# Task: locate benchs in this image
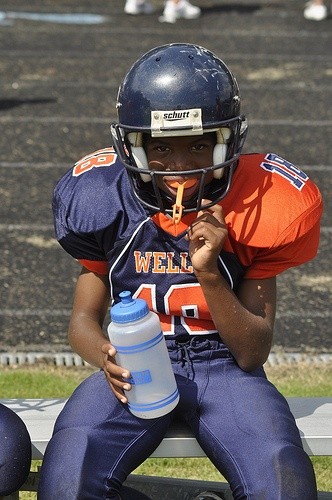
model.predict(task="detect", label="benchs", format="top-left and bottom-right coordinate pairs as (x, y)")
top-left (0, 397), bottom-right (332, 500)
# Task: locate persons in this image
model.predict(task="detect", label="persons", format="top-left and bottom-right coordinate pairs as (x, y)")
top-left (304, 0), bottom-right (328, 20)
top-left (124, 0), bottom-right (201, 19)
top-left (36, 43), bottom-right (323, 500)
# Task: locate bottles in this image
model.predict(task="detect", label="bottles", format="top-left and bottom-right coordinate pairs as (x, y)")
top-left (107, 291), bottom-right (180, 419)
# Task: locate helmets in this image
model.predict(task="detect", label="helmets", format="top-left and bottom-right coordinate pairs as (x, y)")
top-left (110, 42), bottom-right (247, 216)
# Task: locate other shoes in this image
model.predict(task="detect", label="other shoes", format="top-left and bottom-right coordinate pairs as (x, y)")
top-left (163, 1), bottom-right (201, 22)
top-left (303, 4), bottom-right (328, 21)
top-left (123, 0), bottom-right (157, 16)
